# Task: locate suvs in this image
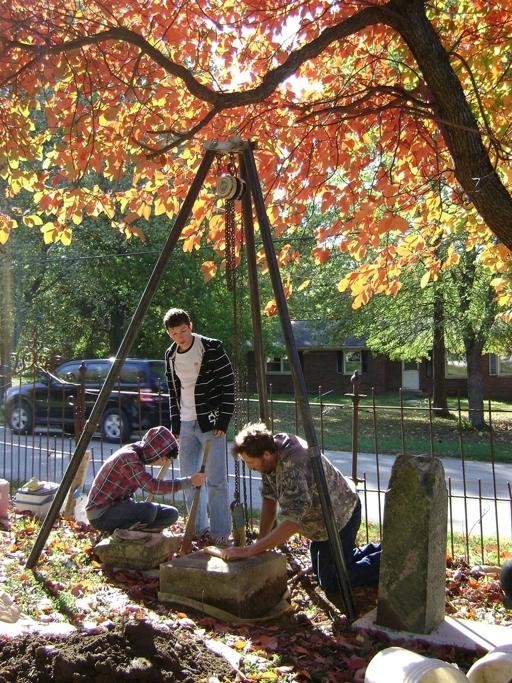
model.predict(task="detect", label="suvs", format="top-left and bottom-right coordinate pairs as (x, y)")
top-left (2, 359), bottom-right (183, 440)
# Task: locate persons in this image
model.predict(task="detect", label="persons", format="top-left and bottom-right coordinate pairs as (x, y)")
top-left (163, 308), bottom-right (234, 546)
top-left (221, 422), bottom-right (381, 593)
top-left (85, 426), bottom-right (207, 540)
